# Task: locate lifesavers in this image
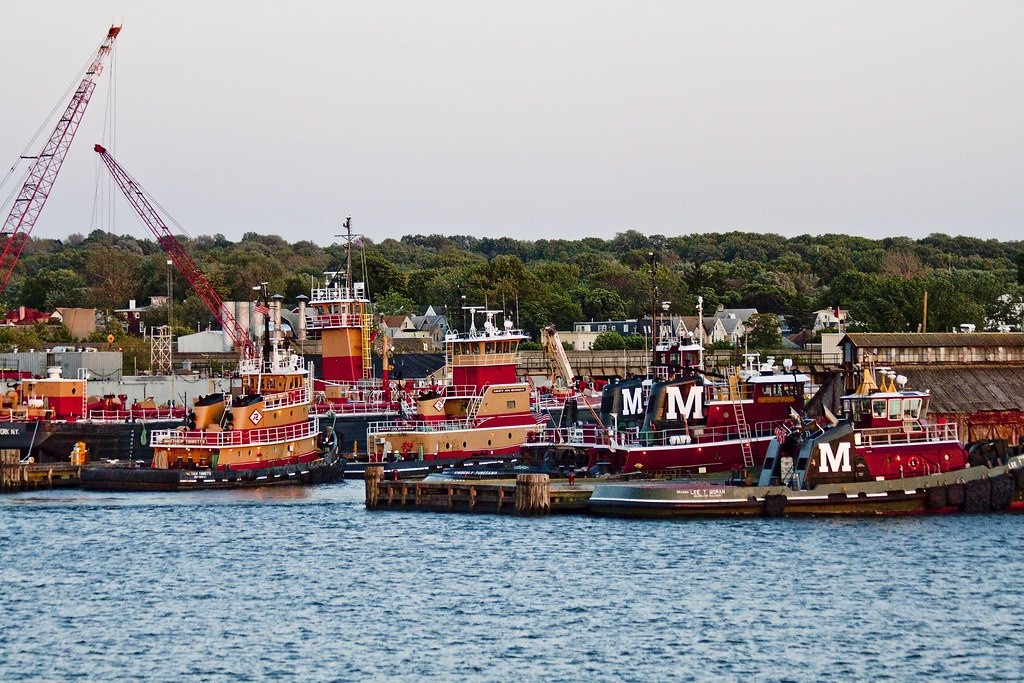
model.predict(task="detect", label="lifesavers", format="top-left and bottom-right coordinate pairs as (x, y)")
top-left (906, 456), bottom-right (920, 470)
top-left (401, 441), bottom-right (411, 452)
top-left (783, 419), bottom-right (794, 428)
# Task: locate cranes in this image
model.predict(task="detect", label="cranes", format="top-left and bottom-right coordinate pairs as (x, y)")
top-left (0, 16), bottom-right (124, 310)
top-left (94, 143), bottom-right (268, 373)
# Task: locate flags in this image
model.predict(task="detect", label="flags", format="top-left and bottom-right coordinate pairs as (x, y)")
top-left (253, 301), bottom-right (270, 314)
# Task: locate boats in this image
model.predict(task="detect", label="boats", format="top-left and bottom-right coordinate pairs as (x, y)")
top-left (0, 206), bottom-right (1024, 519)
